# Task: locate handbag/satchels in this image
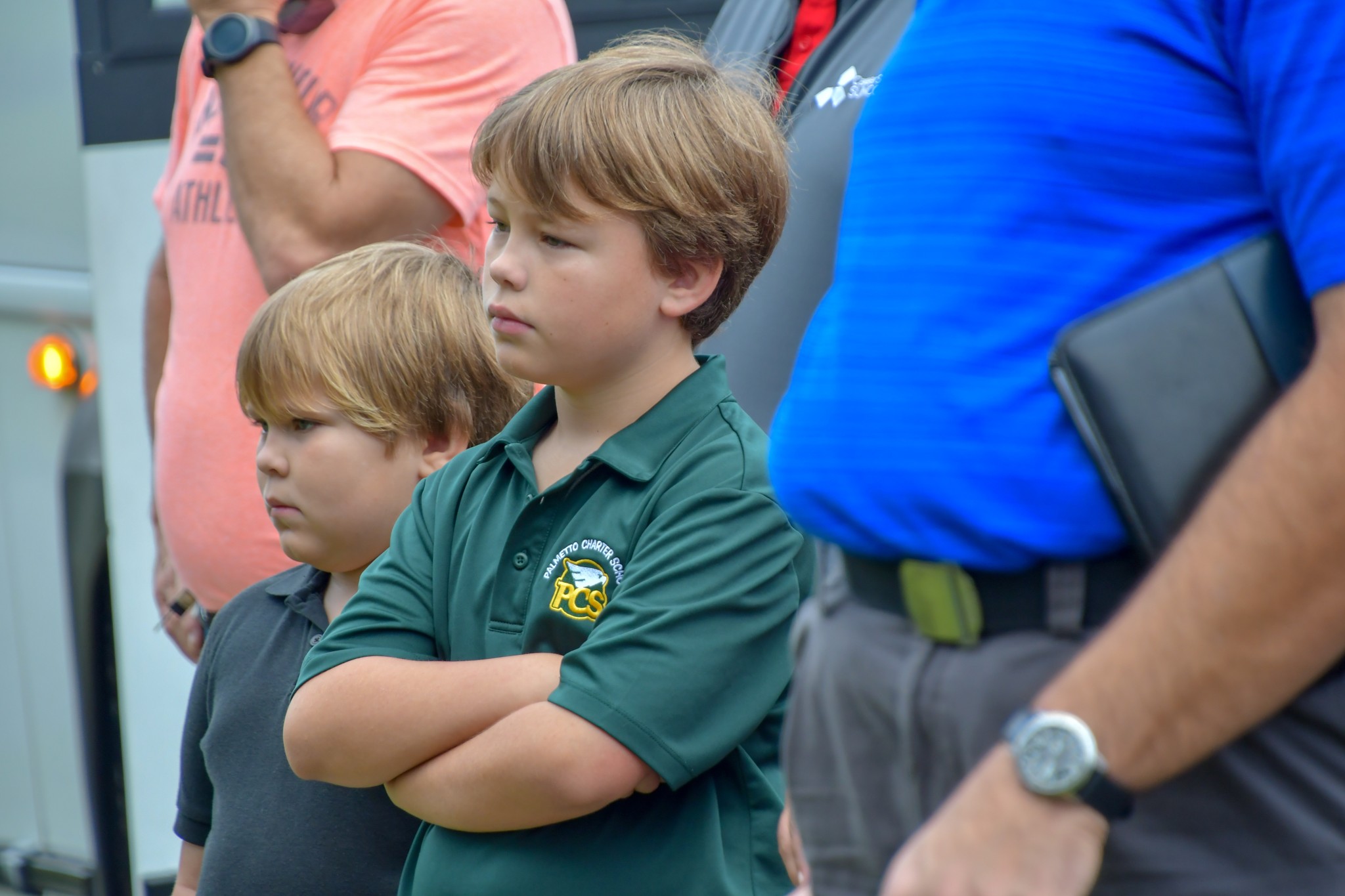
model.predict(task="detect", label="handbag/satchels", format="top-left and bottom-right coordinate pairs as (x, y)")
top-left (1049, 232), bottom-right (1320, 558)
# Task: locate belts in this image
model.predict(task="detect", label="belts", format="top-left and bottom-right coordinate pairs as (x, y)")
top-left (815, 539), bottom-right (1137, 645)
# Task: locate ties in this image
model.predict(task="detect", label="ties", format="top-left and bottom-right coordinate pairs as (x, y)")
top-left (769, 0), bottom-right (836, 118)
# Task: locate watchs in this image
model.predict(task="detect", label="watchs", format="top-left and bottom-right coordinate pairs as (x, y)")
top-left (199, 12), bottom-right (282, 80)
top-left (998, 703), bottom-right (1139, 828)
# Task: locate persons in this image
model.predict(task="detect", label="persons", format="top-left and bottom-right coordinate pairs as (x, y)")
top-left (166, 236), bottom-right (533, 894)
top-left (281, 25), bottom-right (820, 896)
top-left (753, 0), bottom-right (1345, 896)
top-left (136, 0), bottom-right (586, 663)
top-left (686, 0), bottom-right (911, 589)
